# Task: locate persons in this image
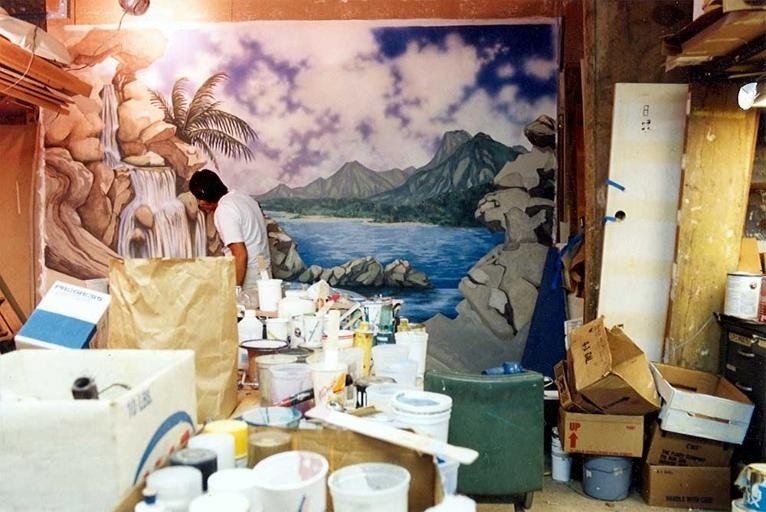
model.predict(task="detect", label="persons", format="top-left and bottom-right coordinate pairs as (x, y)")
top-left (188, 169), bottom-right (273, 289)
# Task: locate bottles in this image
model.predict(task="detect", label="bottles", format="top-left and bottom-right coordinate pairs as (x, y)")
top-left (397, 318), bottom-right (411, 330)
top-left (354, 321), bottom-right (372, 376)
top-left (234, 309), bottom-right (262, 369)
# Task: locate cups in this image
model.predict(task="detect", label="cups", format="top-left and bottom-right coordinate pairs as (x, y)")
top-left (504, 361), bottom-right (520, 375)
top-left (136, 420), bottom-right (411, 511)
top-left (256, 278), bottom-right (283, 310)
top-left (266, 311), bottom-right (341, 344)
top-left (372, 331), bottom-right (452, 389)
top-left (379, 295), bottom-right (392, 333)
top-left (257, 348), bottom-right (348, 407)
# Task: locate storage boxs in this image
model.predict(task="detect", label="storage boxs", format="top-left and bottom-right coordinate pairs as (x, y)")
top-left (556, 316), bottom-right (756, 508)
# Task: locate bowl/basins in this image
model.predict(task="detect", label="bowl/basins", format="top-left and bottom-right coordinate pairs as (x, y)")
top-left (241, 407), bottom-right (302, 430)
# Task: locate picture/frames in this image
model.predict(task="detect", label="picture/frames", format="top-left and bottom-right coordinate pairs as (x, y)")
top-left (45, 2), bottom-right (588, 321)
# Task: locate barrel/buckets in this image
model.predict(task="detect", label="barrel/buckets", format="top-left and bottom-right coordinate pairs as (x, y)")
top-left (724, 271), bottom-right (764, 319)
top-left (582, 455), bottom-right (633, 500)
top-left (253, 277), bottom-right (460, 512)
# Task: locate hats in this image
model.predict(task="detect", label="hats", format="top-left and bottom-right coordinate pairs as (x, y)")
top-left (189, 169), bottom-right (229, 203)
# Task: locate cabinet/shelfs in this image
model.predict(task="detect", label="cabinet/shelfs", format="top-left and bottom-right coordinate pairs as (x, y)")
top-left (712, 312), bottom-right (766, 465)
